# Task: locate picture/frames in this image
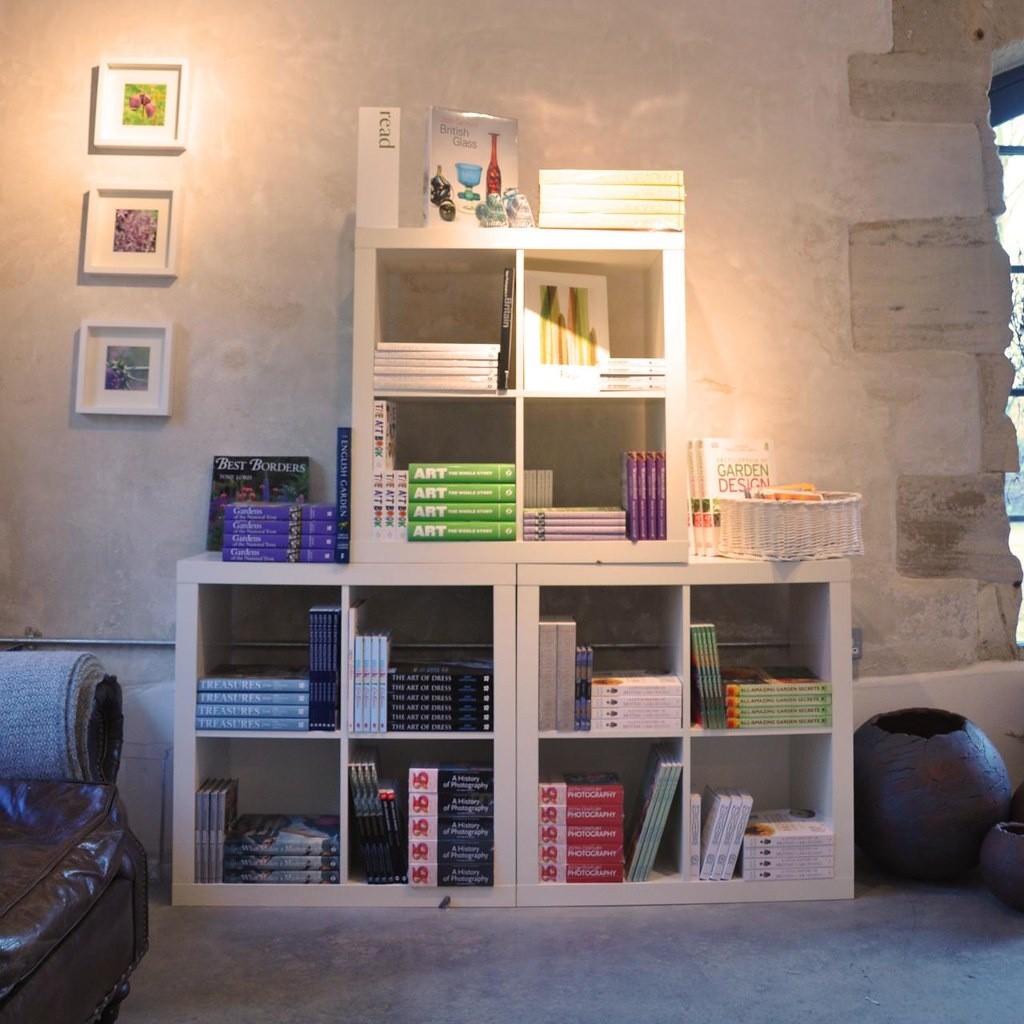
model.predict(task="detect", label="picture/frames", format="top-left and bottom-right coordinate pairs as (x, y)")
top-left (82, 180), bottom-right (180, 278)
top-left (93, 57), bottom-right (190, 152)
top-left (74, 319), bottom-right (173, 416)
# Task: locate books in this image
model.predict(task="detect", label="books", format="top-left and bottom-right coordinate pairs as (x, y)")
top-left (685, 439), bottom-right (779, 557)
top-left (690, 621), bottom-right (833, 730)
top-left (195, 743), bottom-right (684, 886)
top-left (195, 599), bottom-right (681, 733)
top-left (690, 783), bottom-right (835, 882)
top-left (208, 107), bottom-right (684, 566)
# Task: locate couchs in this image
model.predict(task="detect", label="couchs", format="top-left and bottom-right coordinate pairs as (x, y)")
top-left (0, 650), bottom-right (149, 1024)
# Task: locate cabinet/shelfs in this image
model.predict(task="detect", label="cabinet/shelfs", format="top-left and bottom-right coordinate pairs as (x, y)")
top-left (170, 106), bottom-right (854, 907)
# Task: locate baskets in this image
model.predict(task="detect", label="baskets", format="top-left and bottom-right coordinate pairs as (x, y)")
top-left (715, 488), bottom-right (868, 562)
